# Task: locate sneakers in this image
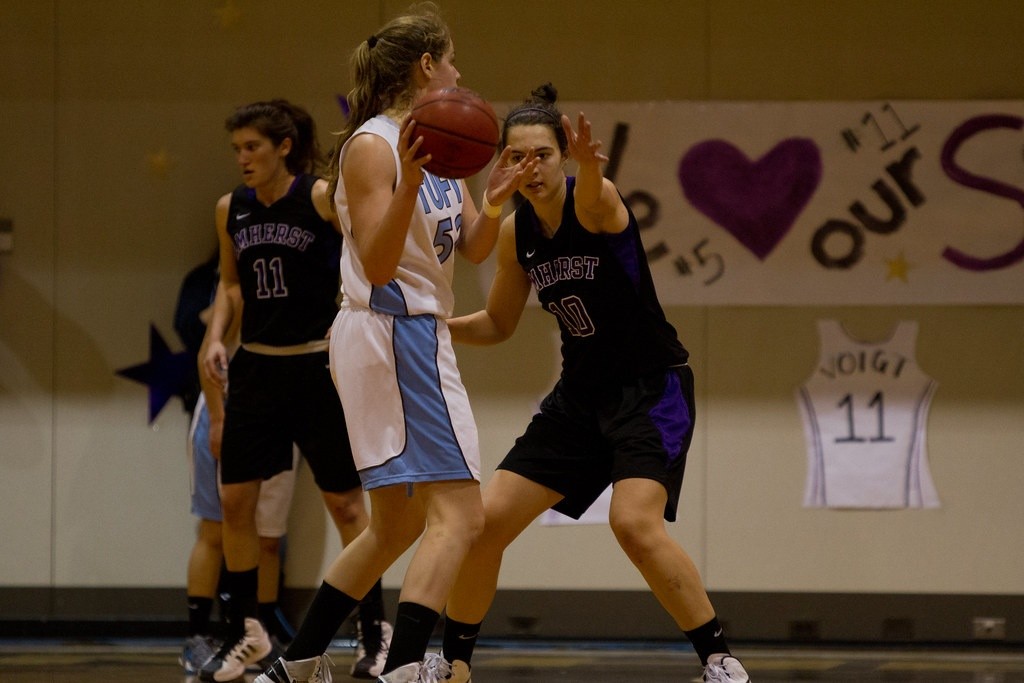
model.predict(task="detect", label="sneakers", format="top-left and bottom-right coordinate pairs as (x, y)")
top-left (377, 648), bottom-right (472, 683)
top-left (214, 617), bottom-right (272, 682)
top-left (253, 650), bottom-right (336, 683)
top-left (347, 612), bottom-right (393, 678)
top-left (177, 635), bottom-right (214, 674)
top-left (700, 653), bottom-right (751, 683)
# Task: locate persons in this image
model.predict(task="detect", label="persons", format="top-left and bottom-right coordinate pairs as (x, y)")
top-left (431, 79), bottom-right (757, 683)
top-left (252, 13), bottom-right (540, 683)
top-left (170, 96), bottom-right (393, 683)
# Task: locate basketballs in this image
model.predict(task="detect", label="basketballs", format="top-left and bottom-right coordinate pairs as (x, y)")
top-left (406, 86), bottom-right (501, 178)
